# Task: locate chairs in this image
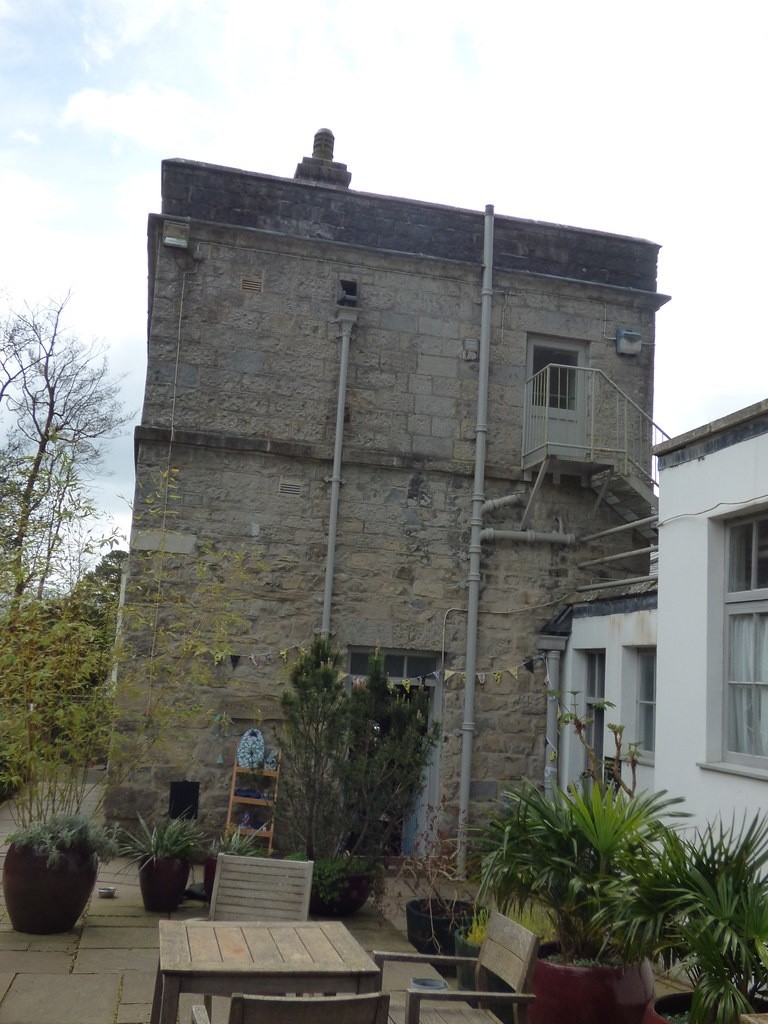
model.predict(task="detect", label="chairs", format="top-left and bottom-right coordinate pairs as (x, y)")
top-left (190, 991), bottom-right (391, 1024)
top-left (371, 908), bottom-right (541, 1024)
top-left (201, 852), bottom-right (315, 1024)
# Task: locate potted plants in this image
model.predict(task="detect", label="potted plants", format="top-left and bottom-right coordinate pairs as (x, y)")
top-left (442, 687), bottom-right (692, 1024)
top-left (450, 894), bottom-right (562, 1024)
top-left (0, 429), bottom-right (312, 931)
top-left (378, 785), bottom-right (488, 979)
top-left (556, 814), bottom-right (768, 1024)
top-left (115, 805), bottom-right (213, 917)
top-left (201, 818), bottom-right (273, 909)
top-left (245, 631), bottom-right (443, 919)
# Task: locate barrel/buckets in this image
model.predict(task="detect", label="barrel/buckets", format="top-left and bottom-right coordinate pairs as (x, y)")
top-left (410, 977), bottom-right (447, 990)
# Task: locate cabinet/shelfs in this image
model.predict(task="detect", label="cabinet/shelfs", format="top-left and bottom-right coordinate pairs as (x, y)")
top-left (223, 743), bottom-right (283, 850)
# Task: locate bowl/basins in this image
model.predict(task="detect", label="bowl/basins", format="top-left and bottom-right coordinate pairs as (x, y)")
top-left (97, 887), bottom-right (117, 898)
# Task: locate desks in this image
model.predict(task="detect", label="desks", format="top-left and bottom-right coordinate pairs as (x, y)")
top-left (151, 919), bottom-right (383, 1024)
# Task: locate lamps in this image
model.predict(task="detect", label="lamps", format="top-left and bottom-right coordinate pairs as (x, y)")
top-left (162, 220), bottom-right (191, 250)
